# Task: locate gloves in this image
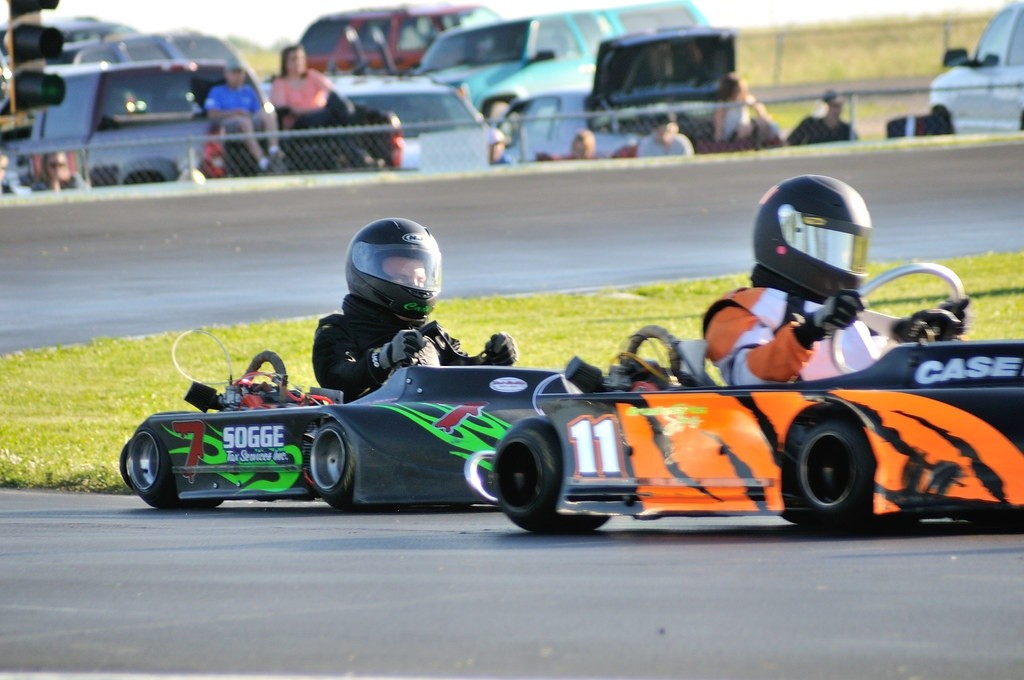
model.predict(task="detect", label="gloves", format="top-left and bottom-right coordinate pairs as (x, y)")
top-left (891, 298), bottom-right (970, 342)
top-left (483, 333), bottom-right (516, 366)
top-left (802, 289), bottom-right (864, 342)
top-left (379, 328), bottom-right (426, 370)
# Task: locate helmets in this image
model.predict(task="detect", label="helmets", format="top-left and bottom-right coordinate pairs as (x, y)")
top-left (345, 217), bottom-right (442, 320)
top-left (752, 174), bottom-right (873, 298)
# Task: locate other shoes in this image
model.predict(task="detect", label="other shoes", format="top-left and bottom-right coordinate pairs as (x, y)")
top-left (263, 161), bottom-right (276, 171)
top-left (270, 150), bottom-right (287, 162)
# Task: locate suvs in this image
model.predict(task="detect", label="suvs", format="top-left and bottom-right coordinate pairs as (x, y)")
top-left (261, 77), bottom-right (508, 169)
top-left (491, 26), bottom-right (786, 167)
top-left (415, 0), bottom-right (712, 120)
top-left (280, 2), bottom-right (503, 72)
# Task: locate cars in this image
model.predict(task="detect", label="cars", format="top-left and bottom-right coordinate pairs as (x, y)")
top-left (44, 17), bottom-right (248, 120)
top-left (928, 0), bottom-right (1023, 132)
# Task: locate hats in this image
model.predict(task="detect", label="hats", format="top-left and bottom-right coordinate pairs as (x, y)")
top-left (484, 126), bottom-right (512, 149)
top-left (226, 58), bottom-right (244, 70)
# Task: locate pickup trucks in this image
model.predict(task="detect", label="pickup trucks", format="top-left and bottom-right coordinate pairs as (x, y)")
top-left (0, 62), bottom-right (274, 190)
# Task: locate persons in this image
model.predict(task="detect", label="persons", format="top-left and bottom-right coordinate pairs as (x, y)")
top-left (30, 147), bottom-right (90, 194)
top-left (702, 173), bottom-right (970, 387)
top-left (313, 216), bottom-right (516, 402)
top-left (451, 76), bottom-right (527, 167)
top-left (532, 119), bottom-right (597, 162)
top-left (635, 112), bottom-right (695, 158)
top-left (714, 74), bottom-right (782, 151)
top-left (786, 88), bottom-right (858, 147)
top-left (206, 43), bottom-right (387, 175)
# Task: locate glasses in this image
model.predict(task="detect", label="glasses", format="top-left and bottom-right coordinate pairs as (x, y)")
top-left (48, 162), bottom-right (66, 169)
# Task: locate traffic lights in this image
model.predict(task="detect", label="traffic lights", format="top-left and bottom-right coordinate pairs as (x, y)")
top-left (8, 0), bottom-right (66, 112)
